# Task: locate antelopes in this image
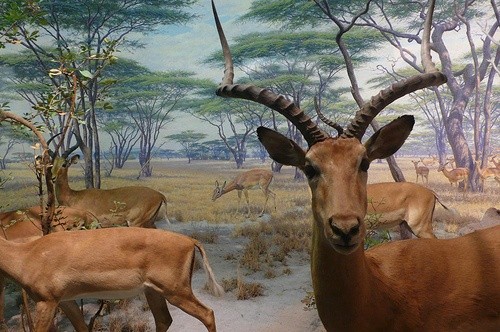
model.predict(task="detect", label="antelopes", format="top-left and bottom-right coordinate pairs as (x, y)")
top-left (364, 183), bottom-right (449, 241)
top-left (410, 152), bottom-right (499, 191)
top-left (211, 1), bottom-right (499, 332)
top-left (0, 228), bottom-right (223, 332)
top-left (33, 131), bottom-right (173, 231)
top-left (0, 205), bottom-right (103, 242)
top-left (212, 169), bottom-right (277, 219)
